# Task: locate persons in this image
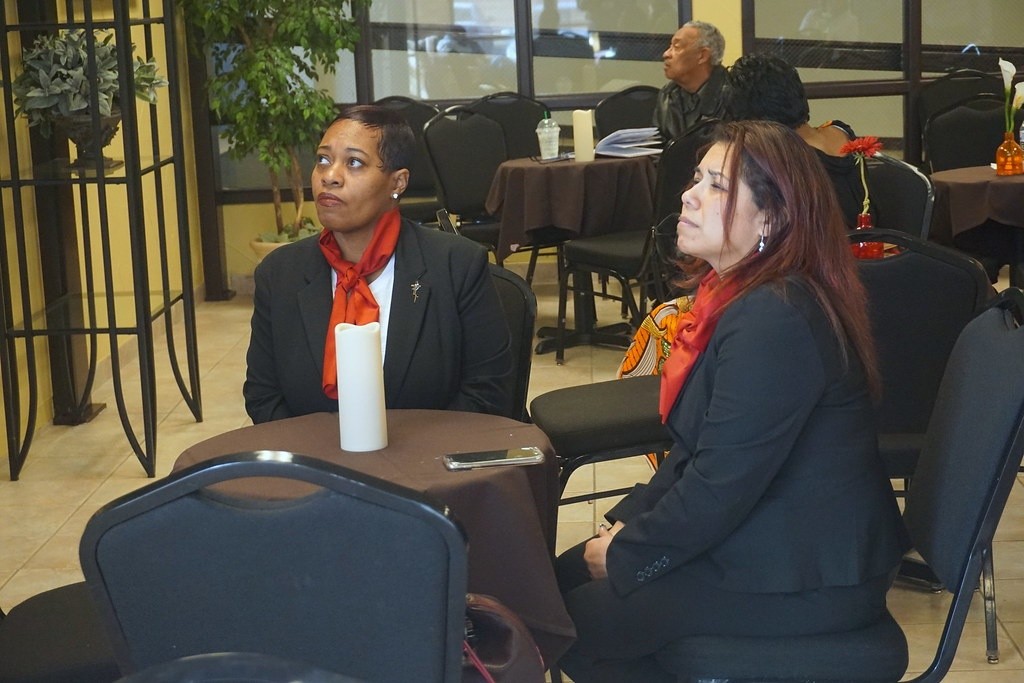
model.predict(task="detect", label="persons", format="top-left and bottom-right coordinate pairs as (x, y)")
top-left (242, 105), bottom-right (516, 416)
top-left (553, 119), bottom-right (910, 683)
top-left (722, 52), bottom-right (878, 228)
top-left (652, 20), bottom-right (730, 144)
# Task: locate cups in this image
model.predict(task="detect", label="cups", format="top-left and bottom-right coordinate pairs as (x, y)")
top-left (534, 119), bottom-right (561, 160)
top-left (571, 109), bottom-right (595, 162)
top-left (334, 321), bottom-right (388, 452)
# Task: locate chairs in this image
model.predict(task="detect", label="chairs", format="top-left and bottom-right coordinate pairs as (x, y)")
top-left (0, 81), bottom-right (1024, 683)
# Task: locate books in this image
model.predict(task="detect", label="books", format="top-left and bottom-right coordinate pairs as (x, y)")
top-left (567, 126), bottom-right (663, 158)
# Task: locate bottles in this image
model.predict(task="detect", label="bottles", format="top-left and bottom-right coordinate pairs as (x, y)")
top-left (1019, 121), bottom-right (1024, 150)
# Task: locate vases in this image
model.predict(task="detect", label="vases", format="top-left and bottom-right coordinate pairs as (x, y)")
top-left (847, 215), bottom-right (884, 261)
top-left (996, 132), bottom-right (1024, 176)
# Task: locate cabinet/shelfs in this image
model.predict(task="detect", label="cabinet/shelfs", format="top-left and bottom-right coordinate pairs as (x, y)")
top-left (0, 0), bottom-right (204, 480)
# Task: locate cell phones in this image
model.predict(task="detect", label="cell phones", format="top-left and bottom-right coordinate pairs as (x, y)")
top-left (443, 447), bottom-right (545, 471)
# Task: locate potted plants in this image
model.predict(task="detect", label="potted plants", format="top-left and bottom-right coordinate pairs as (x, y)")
top-left (13, 33), bottom-right (166, 176)
top-left (188, 0), bottom-right (379, 262)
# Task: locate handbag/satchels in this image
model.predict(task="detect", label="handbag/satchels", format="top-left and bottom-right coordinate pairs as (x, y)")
top-left (463, 591), bottom-right (545, 682)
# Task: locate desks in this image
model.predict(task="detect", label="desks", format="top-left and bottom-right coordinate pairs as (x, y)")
top-left (170, 401), bottom-right (579, 683)
top-left (930, 161), bottom-right (1024, 289)
top-left (486, 149), bottom-right (660, 362)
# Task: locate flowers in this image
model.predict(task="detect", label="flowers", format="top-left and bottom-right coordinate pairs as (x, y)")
top-left (837, 134), bottom-right (885, 214)
top-left (989, 57), bottom-right (1024, 135)
